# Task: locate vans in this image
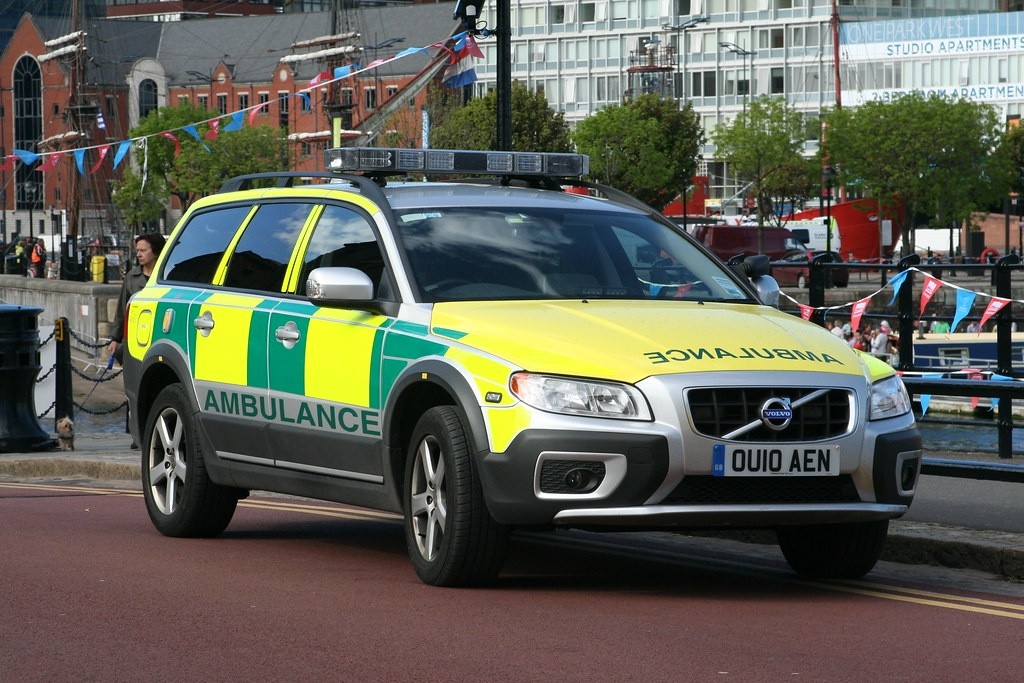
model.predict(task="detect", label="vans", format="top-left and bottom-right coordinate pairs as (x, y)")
top-left (740, 215), bottom-right (843, 261)
top-left (690, 225), bottom-right (815, 289)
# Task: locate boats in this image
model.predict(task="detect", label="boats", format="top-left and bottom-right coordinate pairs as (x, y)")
top-left (889, 330), bottom-right (1024, 420)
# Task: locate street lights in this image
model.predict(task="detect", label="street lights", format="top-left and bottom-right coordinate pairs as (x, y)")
top-left (822, 168), bottom-right (836, 255)
top-left (48, 204), bottom-right (56, 263)
top-left (24, 181), bottom-right (36, 243)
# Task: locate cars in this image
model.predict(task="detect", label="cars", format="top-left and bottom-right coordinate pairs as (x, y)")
top-left (768, 249), bottom-right (849, 287)
top-left (99, 235), bottom-right (132, 251)
top-left (120, 146), bottom-right (925, 589)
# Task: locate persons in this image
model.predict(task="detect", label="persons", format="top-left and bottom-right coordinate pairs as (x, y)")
top-left (105, 233), bottom-right (167, 451)
top-left (823, 311), bottom-right (1020, 373)
top-left (0, 234), bottom-right (132, 278)
